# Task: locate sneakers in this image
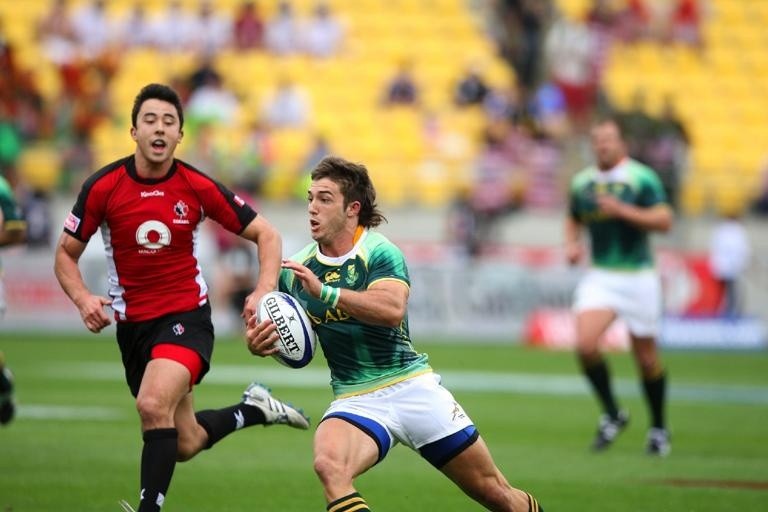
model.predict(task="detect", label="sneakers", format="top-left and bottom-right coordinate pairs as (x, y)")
top-left (591, 407), bottom-right (629, 451)
top-left (242, 379), bottom-right (313, 432)
top-left (644, 425), bottom-right (673, 459)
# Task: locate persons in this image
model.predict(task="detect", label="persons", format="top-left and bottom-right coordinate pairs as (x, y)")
top-left (55, 84), bottom-right (310, 512)
top-left (246, 157), bottom-right (541, 511)
top-left (1, 0), bottom-right (768, 324)
top-left (703, 209), bottom-right (753, 322)
top-left (0, 174), bottom-right (27, 426)
top-left (565, 114), bottom-right (672, 458)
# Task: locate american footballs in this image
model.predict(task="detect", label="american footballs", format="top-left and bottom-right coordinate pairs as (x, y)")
top-left (257, 291), bottom-right (316, 369)
